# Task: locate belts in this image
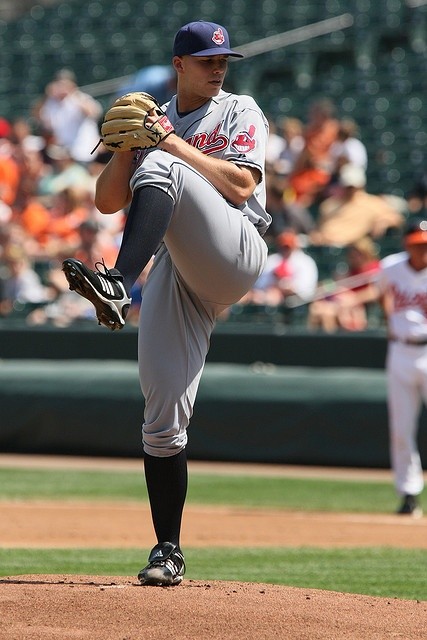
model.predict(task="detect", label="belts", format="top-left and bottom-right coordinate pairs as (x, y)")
top-left (389, 334), bottom-right (426, 348)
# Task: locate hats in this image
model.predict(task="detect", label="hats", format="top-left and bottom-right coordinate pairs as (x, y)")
top-left (277, 231), bottom-right (293, 248)
top-left (174, 18), bottom-right (244, 60)
top-left (403, 217), bottom-right (427, 245)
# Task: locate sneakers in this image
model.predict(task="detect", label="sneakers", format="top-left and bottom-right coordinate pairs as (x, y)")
top-left (137, 541), bottom-right (186, 586)
top-left (61, 258), bottom-right (132, 331)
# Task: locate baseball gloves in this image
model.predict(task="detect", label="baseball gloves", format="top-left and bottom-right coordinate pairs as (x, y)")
top-left (90, 91), bottom-right (175, 154)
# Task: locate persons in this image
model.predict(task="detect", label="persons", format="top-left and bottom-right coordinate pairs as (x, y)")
top-left (337, 220), bottom-right (427, 518)
top-left (245, 106), bottom-right (405, 331)
top-left (64, 17), bottom-right (273, 588)
top-left (0, 71), bottom-right (151, 331)
